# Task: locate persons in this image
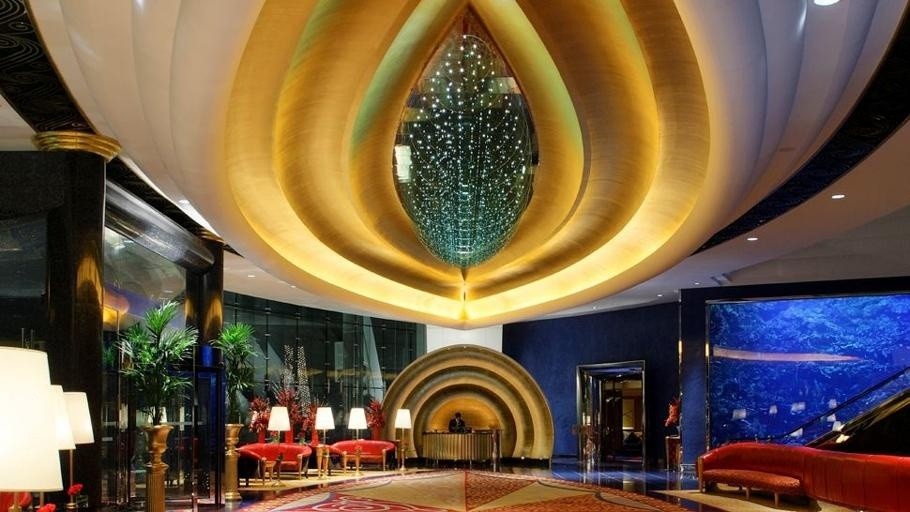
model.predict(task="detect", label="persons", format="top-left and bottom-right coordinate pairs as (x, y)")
top-left (448, 412), bottom-right (465, 434)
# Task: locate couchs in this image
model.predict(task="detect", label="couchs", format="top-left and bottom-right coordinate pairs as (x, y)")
top-left (236, 439), bottom-right (397, 489)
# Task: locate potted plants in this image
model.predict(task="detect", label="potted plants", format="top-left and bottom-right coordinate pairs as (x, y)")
top-left (111, 296), bottom-right (200, 511)
top-left (206, 321), bottom-right (260, 502)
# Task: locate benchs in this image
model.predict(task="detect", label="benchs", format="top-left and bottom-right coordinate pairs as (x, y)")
top-left (702, 468), bottom-right (802, 507)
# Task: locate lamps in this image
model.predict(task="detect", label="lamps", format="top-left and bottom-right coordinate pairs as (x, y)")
top-left (265, 405), bottom-right (412, 488)
